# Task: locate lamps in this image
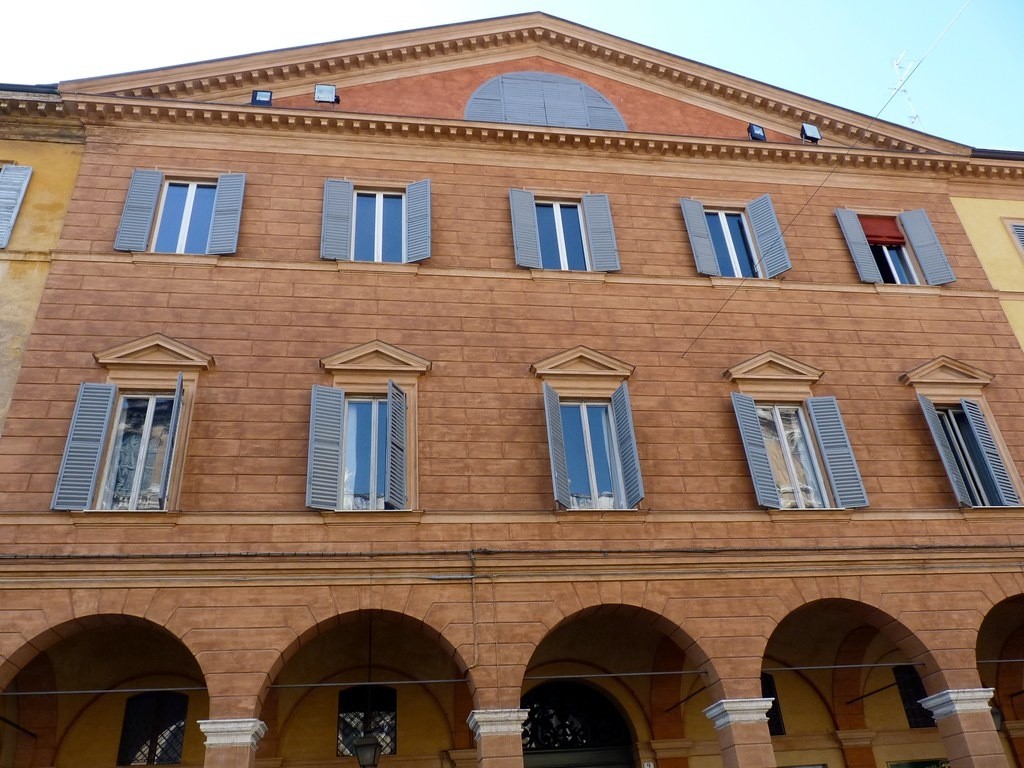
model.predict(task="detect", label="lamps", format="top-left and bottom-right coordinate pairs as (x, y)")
top-left (354, 620), bottom-right (382, 768)
top-left (798, 124), bottom-right (820, 145)
top-left (748, 122), bottom-right (767, 140)
top-left (314, 83), bottom-right (341, 112)
top-left (252, 90), bottom-right (272, 106)
